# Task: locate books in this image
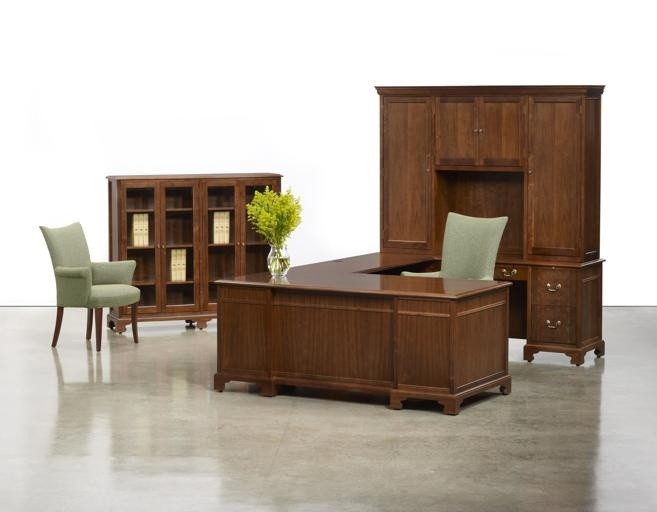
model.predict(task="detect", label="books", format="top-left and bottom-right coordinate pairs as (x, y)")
top-left (170, 249), bottom-right (187, 282)
top-left (131, 213), bottom-right (149, 247)
top-left (213, 211), bottom-right (230, 244)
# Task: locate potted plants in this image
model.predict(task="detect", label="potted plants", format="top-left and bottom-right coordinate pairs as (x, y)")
top-left (245, 184), bottom-right (304, 278)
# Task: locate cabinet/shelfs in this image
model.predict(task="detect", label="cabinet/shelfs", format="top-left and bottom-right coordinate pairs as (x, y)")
top-left (373, 86), bottom-right (606, 366)
top-left (213, 252), bottom-right (514, 415)
top-left (105, 173), bottom-right (284, 335)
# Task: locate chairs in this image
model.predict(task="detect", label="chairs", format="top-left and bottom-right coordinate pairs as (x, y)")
top-left (38, 222), bottom-right (141, 352)
top-left (400, 210), bottom-right (510, 281)
